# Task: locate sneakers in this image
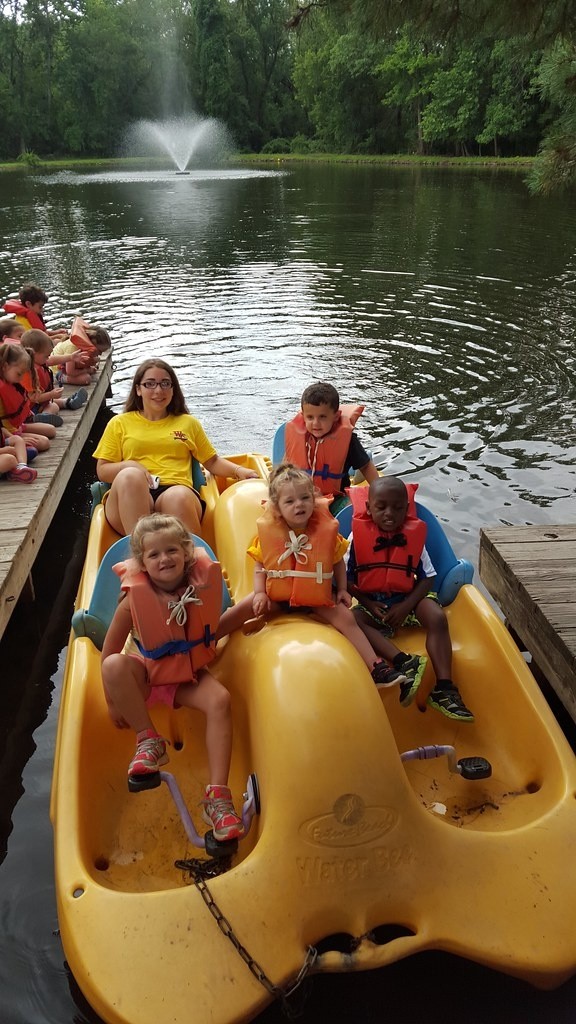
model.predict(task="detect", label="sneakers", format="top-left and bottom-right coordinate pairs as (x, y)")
top-left (425, 685), bottom-right (474, 721)
top-left (127, 729), bottom-right (171, 776)
top-left (198, 784), bottom-right (246, 841)
top-left (371, 658), bottom-right (408, 690)
top-left (396, 654), bottom-right (427, 706)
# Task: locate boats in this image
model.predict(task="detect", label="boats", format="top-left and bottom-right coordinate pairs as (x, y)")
top-left (44, 451), bottom-right (576, 1024)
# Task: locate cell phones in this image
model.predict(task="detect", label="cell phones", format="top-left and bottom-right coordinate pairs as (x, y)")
top-left (148, 475), bottom-right (159, 489)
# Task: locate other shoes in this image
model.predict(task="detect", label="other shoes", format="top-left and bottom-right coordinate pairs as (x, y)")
top-left (53, 370), bottom-right (64, 385)
top-left (26, 447), bottom-right (38, 460)
top-left (65, 387), bottom-right (88, 410)
top-left (6, 465), bottom-right (37, 483)
top-left (33, 413), bottom-right (64, 427)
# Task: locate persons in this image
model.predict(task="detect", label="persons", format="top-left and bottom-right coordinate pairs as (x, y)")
top-left (0, 282), bottom-right (111, 484)
top-left (345, 476), bottom-right (475, 721)
top-left (100, 513), bottom-right (245, 839)
top-left (91, 359), bottom-right (260, 538)
top-left (214, 464), bottom-right (407, 690)
top-left (281, 382), bottom-right (380, 513)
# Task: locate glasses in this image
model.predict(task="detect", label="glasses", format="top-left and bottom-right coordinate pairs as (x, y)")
top-left (139, 381), bottom-right (173, 389)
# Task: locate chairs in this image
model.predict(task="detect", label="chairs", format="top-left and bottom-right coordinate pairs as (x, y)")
top-left (333, 499), bottom-right (473, 607)
top-left (72, 531), bottom-right (234, 652)
top-left (273, 420), bottom-right (374, 487)
